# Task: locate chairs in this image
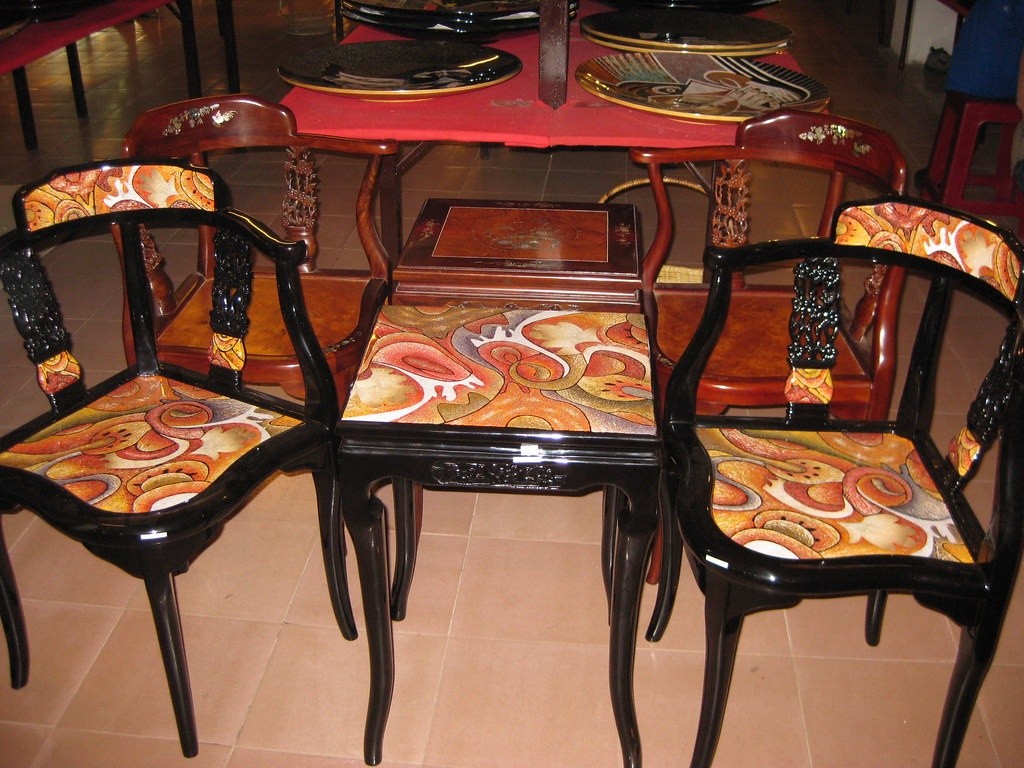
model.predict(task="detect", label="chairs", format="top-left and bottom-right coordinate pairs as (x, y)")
top-left (0, 94), bottom-right (1024, 768)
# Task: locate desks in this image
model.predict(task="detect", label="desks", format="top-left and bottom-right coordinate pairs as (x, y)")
top-left (0, 0), bottom-right (239, 151)
top-left (279, 1), bottom-right (828, 281)
top-left (351, 305), bottom-right (668, 768)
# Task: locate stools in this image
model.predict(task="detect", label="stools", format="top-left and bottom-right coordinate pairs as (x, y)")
top-left (922, 91), bottom-right (1024, 244)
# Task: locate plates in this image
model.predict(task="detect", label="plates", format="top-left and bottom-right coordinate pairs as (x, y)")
top-left (578, 0), bottom-right (793, 56)
top-left (575, 53), bottom-right (832, 123)
top-left (274, 44), bottom-right (523, 103)
top-left (340, 0), bottom-right (581, 42)
